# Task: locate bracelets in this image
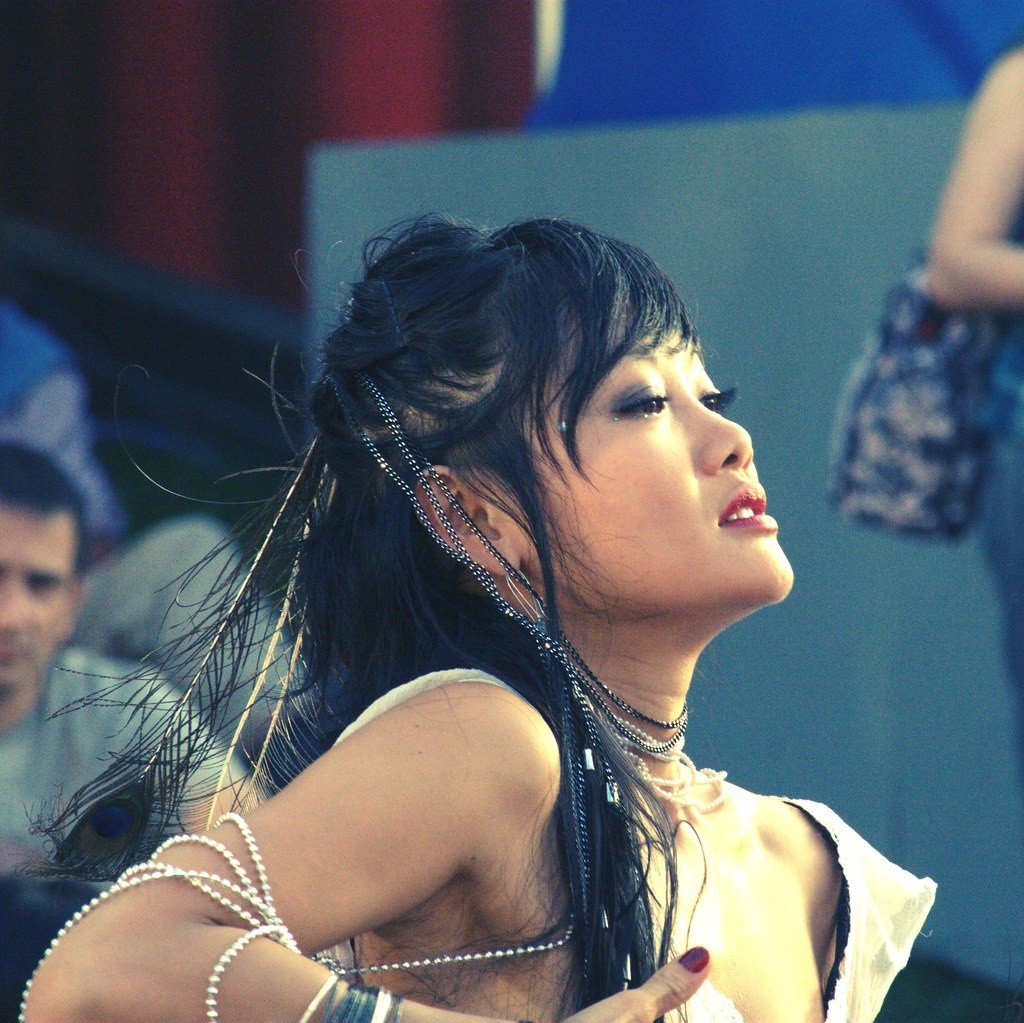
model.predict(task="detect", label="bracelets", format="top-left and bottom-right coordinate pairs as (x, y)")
top-left (364, 984), bottom-right (397, 1021)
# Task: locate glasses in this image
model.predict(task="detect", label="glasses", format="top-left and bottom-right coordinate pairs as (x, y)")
top-left (1, 562), bottom-right (68, 602)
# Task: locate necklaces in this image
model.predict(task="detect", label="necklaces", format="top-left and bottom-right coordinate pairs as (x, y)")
top-left (573, 718), bottom-right (727, 827)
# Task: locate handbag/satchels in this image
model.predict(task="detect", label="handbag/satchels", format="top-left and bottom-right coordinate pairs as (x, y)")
top-left (816, 236), bottom-right (1007, 547)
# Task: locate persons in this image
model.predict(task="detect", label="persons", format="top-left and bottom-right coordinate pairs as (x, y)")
top-left (1, 440), bottom-right (270, 870)
top-left (835, 35), bottom-right (1021, 778)
top-left (4, 294), bottom-right (118, 575)
top-left (22, 209), bottom-right (938, 1023)
top-left (6, 879), bottom-right (100, 1020)
